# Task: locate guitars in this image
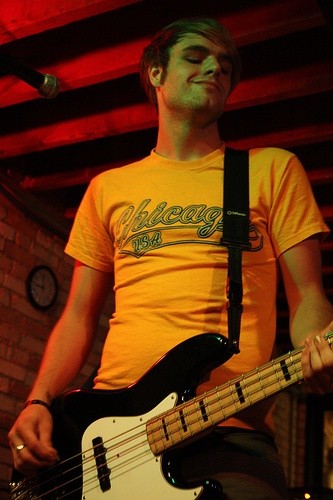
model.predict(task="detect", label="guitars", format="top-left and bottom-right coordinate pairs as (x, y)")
top-left (8, 331), bottom-right (333, 500)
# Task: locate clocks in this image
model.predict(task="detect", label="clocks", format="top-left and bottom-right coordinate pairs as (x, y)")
top-left (26, 264), bottom-right (58, 312)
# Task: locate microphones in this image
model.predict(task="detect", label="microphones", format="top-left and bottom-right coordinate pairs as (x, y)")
top-left (0, 53), bottom-right (60, 101)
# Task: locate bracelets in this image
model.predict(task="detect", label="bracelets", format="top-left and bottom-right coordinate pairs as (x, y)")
top-left (25, 400), bottom-right (49, 410)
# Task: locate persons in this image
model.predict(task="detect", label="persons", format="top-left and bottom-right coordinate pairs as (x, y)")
top-left (8, 18), bottom-right (332, 500)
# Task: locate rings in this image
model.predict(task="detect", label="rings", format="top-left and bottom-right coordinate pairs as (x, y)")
top-left (16, 444), bottom-right (27, 453)
